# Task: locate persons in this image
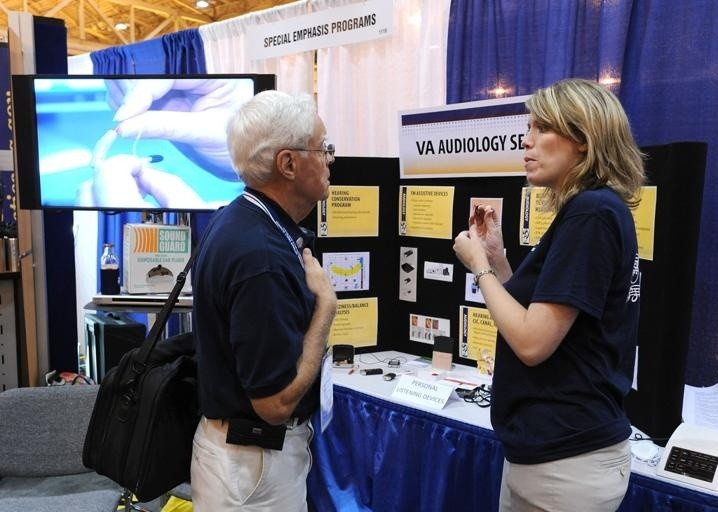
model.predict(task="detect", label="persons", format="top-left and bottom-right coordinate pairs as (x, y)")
top-left (452, 77), bottom-right (642, 510)
top-left (77, 77), bottom-right (254, 209)
top-left (190, 90), bottom-right (339, 512)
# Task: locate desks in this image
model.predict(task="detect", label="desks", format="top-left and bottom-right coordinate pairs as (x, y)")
top-left (311, 348), bottom-right (718, 512)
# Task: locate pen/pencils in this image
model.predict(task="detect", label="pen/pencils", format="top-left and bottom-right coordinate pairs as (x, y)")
top-left (348, 364), bottom-right (359, 374)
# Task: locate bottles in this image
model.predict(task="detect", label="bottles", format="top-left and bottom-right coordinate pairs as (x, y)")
top-left (100, 244), bottom-right (121, 295)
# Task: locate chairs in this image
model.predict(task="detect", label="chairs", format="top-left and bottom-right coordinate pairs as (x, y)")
top-left (0, 384), bottom-right (123, 512)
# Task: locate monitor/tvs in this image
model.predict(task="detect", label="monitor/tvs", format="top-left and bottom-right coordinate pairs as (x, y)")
top-left (12, 73), bottom-right (276, 212)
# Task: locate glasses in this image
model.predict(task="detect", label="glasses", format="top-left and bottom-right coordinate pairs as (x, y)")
top-left (276, 143), bottom-right (335, 162)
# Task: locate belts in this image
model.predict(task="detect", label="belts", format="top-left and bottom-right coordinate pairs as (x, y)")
top-left (288, 415), bottom-right (309, 427)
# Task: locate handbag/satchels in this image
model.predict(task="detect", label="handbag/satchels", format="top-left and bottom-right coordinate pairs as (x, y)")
top-left (81, 331), bottom-right (200, 503)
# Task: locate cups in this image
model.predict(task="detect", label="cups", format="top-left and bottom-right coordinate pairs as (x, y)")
top-left (332, 344), bottom-right (355, 368)
top-left (0, 235), bottom-right (21, 272)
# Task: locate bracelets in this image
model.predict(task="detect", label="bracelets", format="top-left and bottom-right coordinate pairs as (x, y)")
top-left (474, 270), bottom-right (496, 286)
